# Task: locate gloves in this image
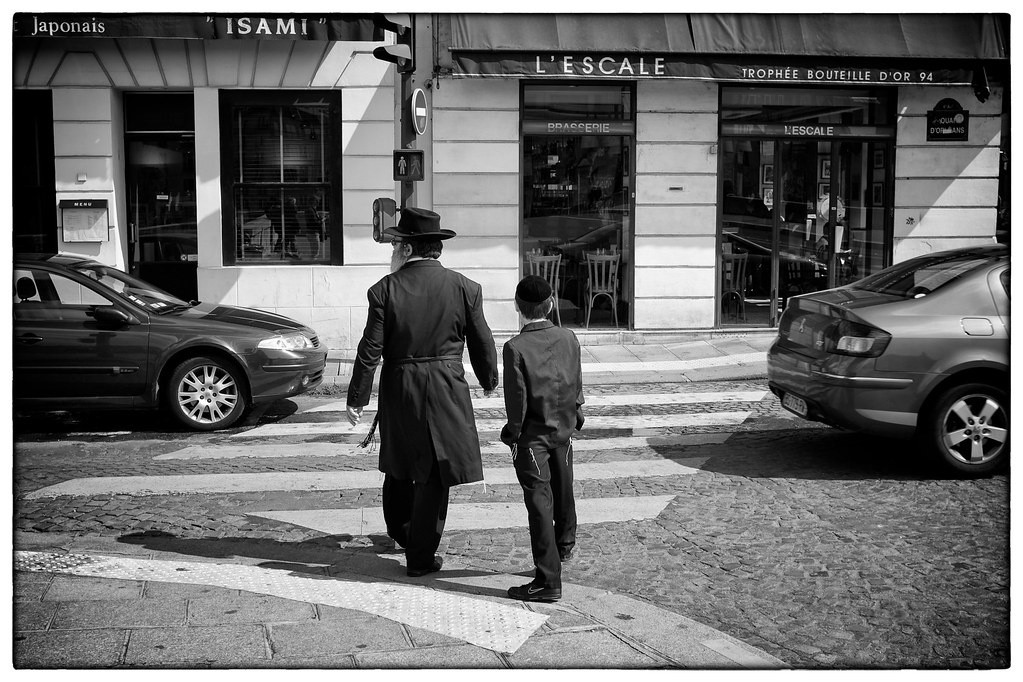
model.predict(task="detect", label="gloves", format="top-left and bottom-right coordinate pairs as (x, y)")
top-left (484, 387), bottom-right (497, 397)
top-left (346, 405), bottom-right (364, 427)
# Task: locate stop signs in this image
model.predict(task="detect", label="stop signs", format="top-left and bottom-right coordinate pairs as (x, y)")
top-left (412, 87), bottom-right (428, 135)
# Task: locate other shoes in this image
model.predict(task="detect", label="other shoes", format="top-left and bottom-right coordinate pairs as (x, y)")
top-left (407, 556), bottom-right (443, 577)
top-left (387, 527), bottom-right (407, 548)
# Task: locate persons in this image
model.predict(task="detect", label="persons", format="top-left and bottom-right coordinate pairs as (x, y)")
top-left (501, 275), bottom-right (584, 602)
top-left (346, 207), bottom-right (498, 576)
top-left (267, 196), bottom-right (324, 260)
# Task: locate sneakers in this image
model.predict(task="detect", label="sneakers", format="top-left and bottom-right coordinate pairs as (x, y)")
top-left (560, 551), bottom-right (573, 562)
top-left (508, 581), bottom-right (562, 602)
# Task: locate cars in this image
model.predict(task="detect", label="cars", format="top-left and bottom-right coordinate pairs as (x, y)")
top-left (765, 239), bottom-right (1012, 473)
top-left (536, 220), bottom-right (832, 325)
top-left (127, 230), bottom-right (269, 263)
top-left (12, 250), bottom-right (330, 432)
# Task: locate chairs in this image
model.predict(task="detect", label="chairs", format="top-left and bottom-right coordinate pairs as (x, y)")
top-left (16, 277), bottom-right (49, 321)
top-left (779, 248), bottom-right (860, 313)
top-left (526, 254), bottom-right (563, 329)
top-left (582, 248), bottom-right (620, 331)
top-left (721, 253), bottom-right (748, 324)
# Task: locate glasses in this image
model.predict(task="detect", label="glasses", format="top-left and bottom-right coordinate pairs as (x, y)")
top-left (390, 238), bottom-right (404, 246)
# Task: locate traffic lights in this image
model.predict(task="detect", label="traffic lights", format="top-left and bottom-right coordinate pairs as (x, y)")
top-left (372, 13), bottom-right (414, 73)
top-left (392, 149), bottom-right (425, 181)
top-left (373, 197), bottom-right (395, 243)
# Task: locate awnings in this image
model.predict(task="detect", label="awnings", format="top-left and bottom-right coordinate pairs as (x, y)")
top-left (451, 13), bottom-right (1008, 85)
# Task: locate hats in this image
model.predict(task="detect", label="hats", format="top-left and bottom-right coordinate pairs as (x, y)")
top-left (381, 207), bottom-right (456, 239)
top-left (517, 275), bottom-right (552, 302)
